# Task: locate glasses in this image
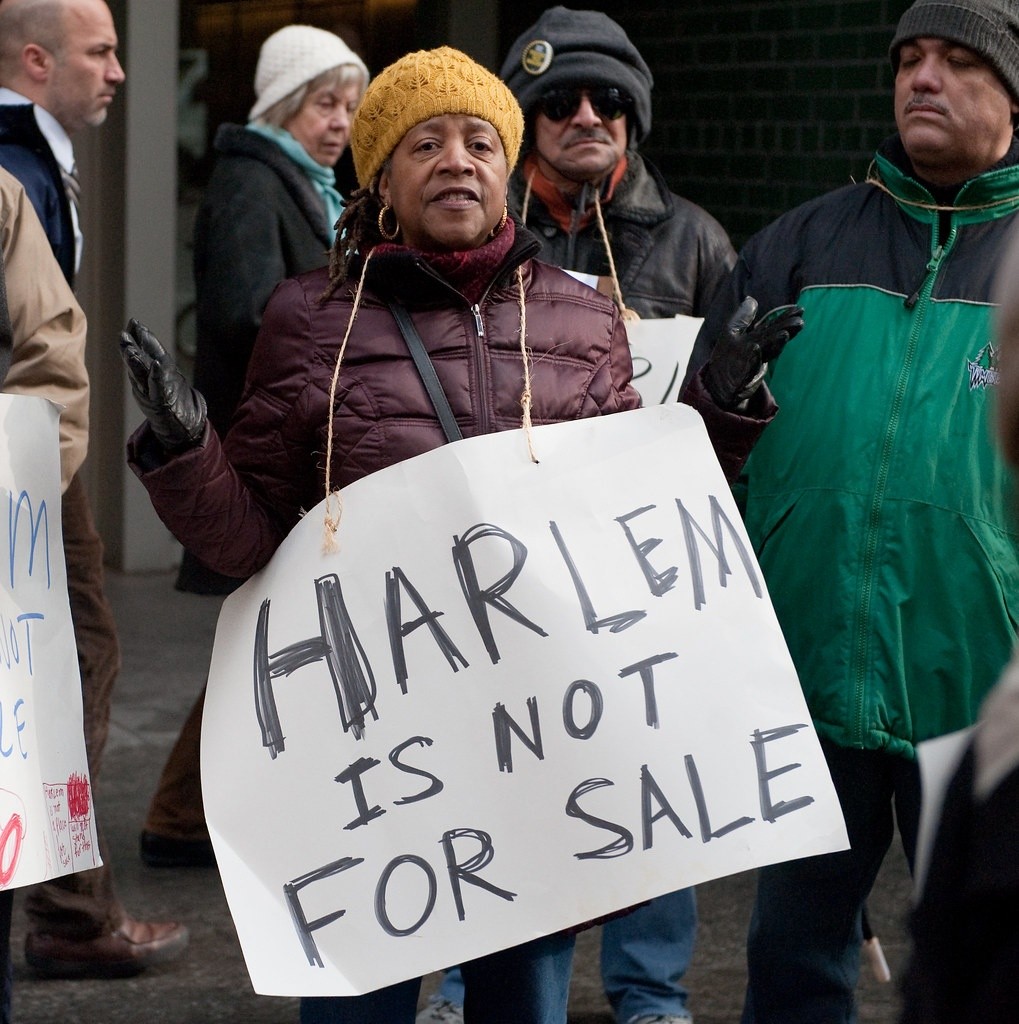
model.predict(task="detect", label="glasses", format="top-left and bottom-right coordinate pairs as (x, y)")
top-left (538, 83), bottom-right (634, 120)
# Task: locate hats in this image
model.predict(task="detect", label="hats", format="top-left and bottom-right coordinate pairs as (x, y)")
top-left (500, 7), bottom-right (655, 150)
top-left (248, 25), bottom-right (369, 121)
top-left (351, 46), bottom-right (524, 190)
top-left (889, 0), bottom-right (1019, 102)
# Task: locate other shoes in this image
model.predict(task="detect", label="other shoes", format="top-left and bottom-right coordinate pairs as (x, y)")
top-left (26, 916), bottom-right (188, 979)
top-left (141, 831), bottom-right (218, 869)
top-left (413, 995), bottom-right (464, 1024)
top-left (632, 1015), bottom-right (693, 1024)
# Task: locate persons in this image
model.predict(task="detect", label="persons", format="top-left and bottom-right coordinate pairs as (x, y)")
top-left (134, 22), bottom-right (371, 868)
top-left (500, 0), bottom-right (756, 1023)
top-left (678, 0), bottom-right (1019, 1023)
top-left (1, 167), bottom-right (92, 1023)
top-left (118, 47), bottom-right (645, 1022)
top-left (0, 0), bottom-right (188, 982)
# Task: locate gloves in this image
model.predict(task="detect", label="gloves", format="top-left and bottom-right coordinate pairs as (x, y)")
top-left (116, 318), bottom-right (207, 450)
top-left (702, 296), bottom-right (804, 413)
